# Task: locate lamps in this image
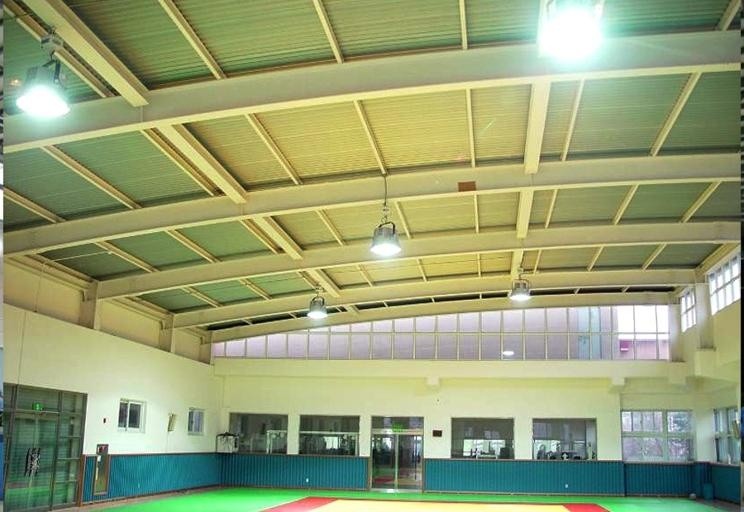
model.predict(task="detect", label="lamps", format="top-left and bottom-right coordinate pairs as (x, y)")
top-left (507, 265), bottom-right (533, 303)
top-left (12, 24), bottom-right (69, 124)
top-left (307, 284), bottom-right (328, 320)
top-left (368, 172), bottom-right (404, 257)
